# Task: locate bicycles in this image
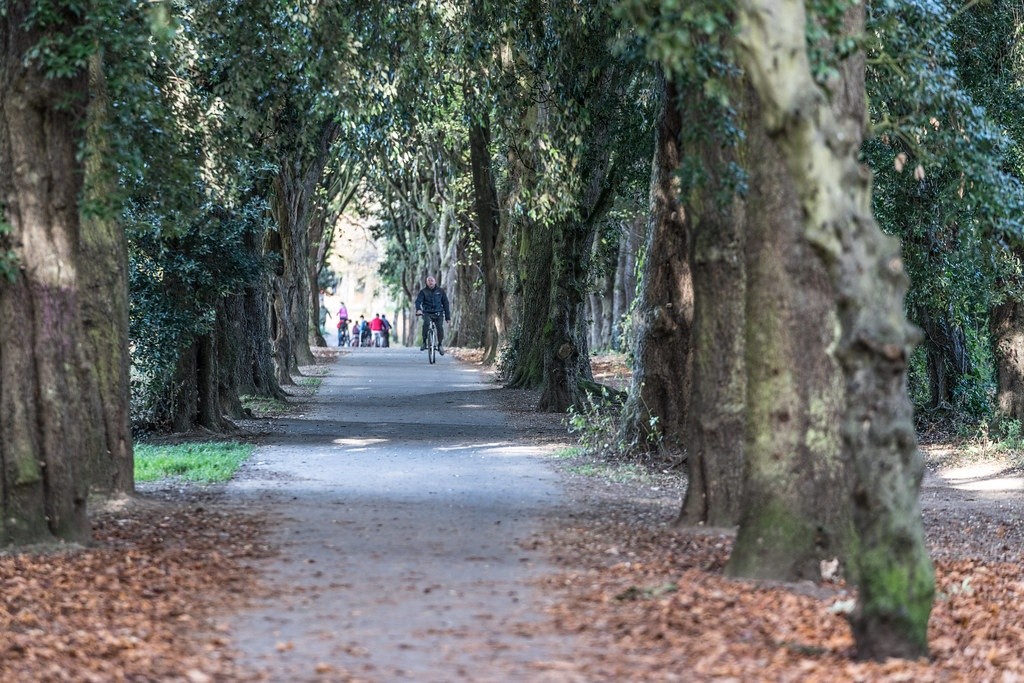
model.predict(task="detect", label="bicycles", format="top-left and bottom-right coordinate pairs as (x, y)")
top-left (339, 332), bottom-right (390, 347)
top-left (415, 312), bottom-right (446, 364)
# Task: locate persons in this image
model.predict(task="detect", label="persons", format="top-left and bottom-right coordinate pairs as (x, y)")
top-left (319, 305), bottom-right (332, 330)
top-left (336, 302), bottom-right (348, 321)
top-left (416, 276), bottom-right (451, 355)
top-left (337, 314), bottom-right (392, 347)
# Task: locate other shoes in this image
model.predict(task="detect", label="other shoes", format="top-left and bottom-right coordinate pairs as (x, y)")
top-left (438, 344), bottom-right (444, 355)
top-left (420, 343), bottom-right (427, 350)
